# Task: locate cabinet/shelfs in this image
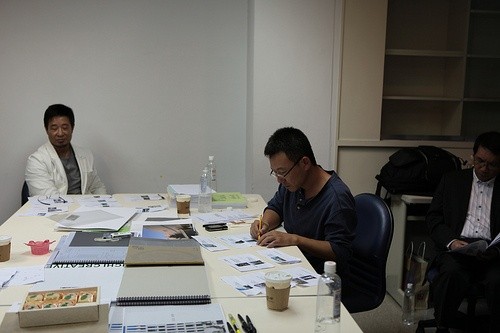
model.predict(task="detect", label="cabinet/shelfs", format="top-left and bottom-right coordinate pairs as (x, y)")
top-left (335, 0), bottom-right (500, 303)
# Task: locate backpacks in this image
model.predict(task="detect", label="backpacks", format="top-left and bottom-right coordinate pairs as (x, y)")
top-left (376, 145), bottom-right (468, 208)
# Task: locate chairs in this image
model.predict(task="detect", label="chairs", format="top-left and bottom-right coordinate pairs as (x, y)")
top-left (330, 192), bottom-right (395, 313)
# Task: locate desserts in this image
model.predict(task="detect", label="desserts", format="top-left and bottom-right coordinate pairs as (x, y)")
top-left (22, 290), bottom-right (96, 309)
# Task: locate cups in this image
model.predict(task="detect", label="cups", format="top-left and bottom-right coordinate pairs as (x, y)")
top-left (0, 234), bottom-right (12, 262)
top-left (264, 271), bottom-right (293, 311)
top-left (175, 194), bottom-right (191, 216)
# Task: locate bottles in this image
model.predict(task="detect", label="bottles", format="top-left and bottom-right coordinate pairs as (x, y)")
top-left (315, 261), bottom-right (342, 333)
top-left (206, 156), bottom-right (216, 193)
top-left (401, 283), bottom-right (415, 325)
top-left (198, 169), bottom-right (212, 213)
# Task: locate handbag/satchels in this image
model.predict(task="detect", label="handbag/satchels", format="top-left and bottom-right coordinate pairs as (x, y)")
top-left (404, 241), bottom-right (428, 291)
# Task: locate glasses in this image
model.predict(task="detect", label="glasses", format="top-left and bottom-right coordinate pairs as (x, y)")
top-left (270, 156), bottom-right (303, 179)
top-left (476, 154), bottom-right (500, 170)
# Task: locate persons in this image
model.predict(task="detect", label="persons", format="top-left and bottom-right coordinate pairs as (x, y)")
top-left (25, 104), bottom-right (107, 198)
top-left (249, 127), bottom-right (371, 302)
top-left (429, 131), bottom-right (500, 333)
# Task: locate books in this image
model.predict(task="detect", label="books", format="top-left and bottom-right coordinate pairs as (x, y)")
top-left (53, 231), bottom-right (229, 333)
top-left (461, 231), bottom-right (500, 253)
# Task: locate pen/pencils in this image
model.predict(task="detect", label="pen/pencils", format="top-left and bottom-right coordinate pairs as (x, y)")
top-left (158, 194), bottom-right (165, 199)
top-left (245, 314), bottom-right (258, 333)
top-left (227, 322), bottom-right (236, 333)
top-left (238, 314), bottom-right (253, 333)
top-left (228, 314), bottom-right (241, 333)
top-left (257, 214), bottom-right (262, 240)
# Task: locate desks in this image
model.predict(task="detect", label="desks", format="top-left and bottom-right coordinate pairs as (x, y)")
top-left (0, 194), bottom-right (365, 333)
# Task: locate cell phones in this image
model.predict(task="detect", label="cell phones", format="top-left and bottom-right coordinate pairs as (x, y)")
top-left (205, 222), bottom-right (228, 231)
